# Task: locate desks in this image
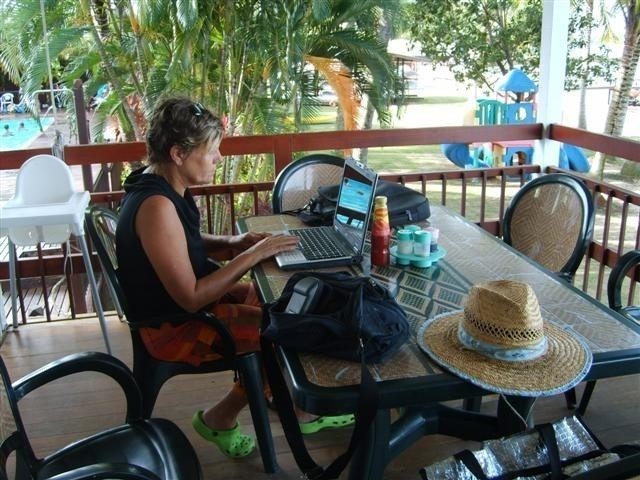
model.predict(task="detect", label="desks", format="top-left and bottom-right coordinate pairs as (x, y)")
top-left (232, 199), bottom-right (640, 477)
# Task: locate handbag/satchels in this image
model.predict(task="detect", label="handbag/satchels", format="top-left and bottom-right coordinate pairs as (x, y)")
top-left (296, 175), bottom-right (433, 231)
top-left (419, 413), bottom-right (622, 480)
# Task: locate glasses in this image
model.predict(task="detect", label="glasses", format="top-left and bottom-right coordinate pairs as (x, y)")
top-left (189, 99), bottom-right (207, 126)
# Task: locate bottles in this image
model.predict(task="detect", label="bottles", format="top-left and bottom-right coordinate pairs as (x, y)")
top-left (370, 195), bottom-right (390, 264)
top-left (399, 224), bottom-right (439, 258)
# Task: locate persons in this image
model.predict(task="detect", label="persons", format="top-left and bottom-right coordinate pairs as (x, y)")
top-left (114, 97), bottom-right (358, 459)
top-left (2, 124), bottom-right (15, 137)
top-left (18, 123), bottom-right (25, 130)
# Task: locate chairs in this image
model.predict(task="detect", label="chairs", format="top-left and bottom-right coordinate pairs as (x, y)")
top-left (270, 152), bottom-right (348, 210)
top-left (0, 153), bottom-right (125, 360)
top-left (0, 92), bottom-right (14, 112)
top-left (0, 347), bottom-right (205, 479)
top-left (500, 173), bottom-right (595, 411)
top-left (576, 249), bottom-right (639, 414)
top-left (85, 200), bottom-right (281, 475)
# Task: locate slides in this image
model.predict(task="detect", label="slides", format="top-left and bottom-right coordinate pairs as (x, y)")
top-left (440, 143), bottom-right (491, 168)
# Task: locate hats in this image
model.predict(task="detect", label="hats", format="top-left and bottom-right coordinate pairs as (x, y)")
top-left (416, 278), bottom-right (594, 398)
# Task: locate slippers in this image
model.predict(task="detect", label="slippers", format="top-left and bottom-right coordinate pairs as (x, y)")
top-left (297, 414), bottom-right (355, 435)
top-left (191, 409), bottom-right (256, 459)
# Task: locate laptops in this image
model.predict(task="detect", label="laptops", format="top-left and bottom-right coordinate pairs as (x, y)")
top-left (264, 155), bottom-right (379, 269)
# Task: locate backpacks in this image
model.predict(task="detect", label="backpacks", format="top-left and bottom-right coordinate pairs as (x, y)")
top-left (259, 271), bottom-right (410, 367)
